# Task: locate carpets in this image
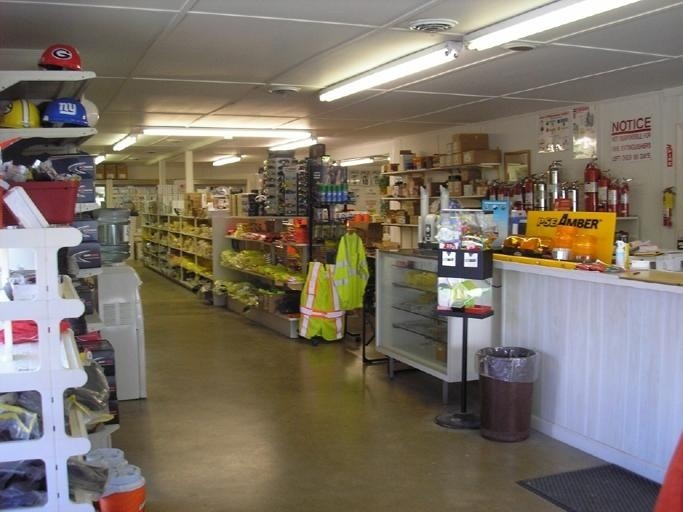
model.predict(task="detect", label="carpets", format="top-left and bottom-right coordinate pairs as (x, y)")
top-left (517, 464), bottom-right (662, 512)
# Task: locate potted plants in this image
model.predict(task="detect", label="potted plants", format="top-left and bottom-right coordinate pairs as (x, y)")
top-left (504, 150), bottom-right (530, 186)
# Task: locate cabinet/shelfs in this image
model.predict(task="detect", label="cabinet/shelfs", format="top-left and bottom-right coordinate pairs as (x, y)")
top-left (376, 248), bottom-right (494, 405)
top-left (614, 233), bottom-right (630, 270)
top-left (94, 207), bottom-right (132, 265)
top-left (141, 210), bottom-right (312, 339)
top-left (313, 182), bottom-right (350, 203)
top-left (0, 71), bottom-right (103, 512)
top-left (381, 162), bottom-right (505, 228)
top-left (446, 175), bottom-right (492, 197)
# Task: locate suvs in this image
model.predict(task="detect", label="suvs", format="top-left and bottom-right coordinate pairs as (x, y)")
top-left (87, 448), bottom-right (146, 512)
top-left (94, 208), bottom-right (130, 263)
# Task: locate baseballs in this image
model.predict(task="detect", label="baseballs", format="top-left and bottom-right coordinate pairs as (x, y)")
top-left (665, 144), bottom-right (673, 167)
top-left (661, 186), bottom-right (676, 227)
top-left (488, 158), bottom-right (633, 217)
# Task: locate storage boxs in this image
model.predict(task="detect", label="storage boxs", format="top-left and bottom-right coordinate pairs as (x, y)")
top-left (439, 133), bottom-right (498, 168)
top-left (94, 162), bottom-right (128, 180)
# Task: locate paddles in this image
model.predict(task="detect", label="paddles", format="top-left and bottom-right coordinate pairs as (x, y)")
top-left (517, 464), bottom-right (662, 512)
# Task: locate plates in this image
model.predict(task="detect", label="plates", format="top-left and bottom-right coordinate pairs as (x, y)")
top-left (381, 162), bottom-right (505, 228)
top-left (376, 248), bottom-right (494, 405)
top-left (141, 210), bottom-right (312, 339)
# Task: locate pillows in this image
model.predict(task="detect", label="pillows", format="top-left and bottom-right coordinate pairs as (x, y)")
top-left (79, 94), bottom-right (100, 128)
top-left (35, 101), bottom-right (57, 128)
top-left (43, 96), bottom-right (88, 127)
top-left (38, 44), bottom-right (82, 71)
top-left (0, 98), bottom-right (41, 128)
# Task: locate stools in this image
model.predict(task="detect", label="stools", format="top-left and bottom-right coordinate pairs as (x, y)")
top-left (435, 305), bottom-right (493, 430)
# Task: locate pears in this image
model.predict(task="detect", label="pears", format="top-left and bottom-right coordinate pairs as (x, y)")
top-left (615, 239), bottom-right (628, 265)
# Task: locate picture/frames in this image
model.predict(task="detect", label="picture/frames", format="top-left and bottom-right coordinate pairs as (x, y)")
top-left (504, 150), bottom-right (530, 186)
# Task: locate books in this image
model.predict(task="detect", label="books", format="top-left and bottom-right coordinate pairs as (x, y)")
top-left (94, 162), bottom-right (128, 180)
top-left (439, 133), bottom-right (498, 168)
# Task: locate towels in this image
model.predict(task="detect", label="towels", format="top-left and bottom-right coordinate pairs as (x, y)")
top-left (475, 347), bottom-right (537, 442)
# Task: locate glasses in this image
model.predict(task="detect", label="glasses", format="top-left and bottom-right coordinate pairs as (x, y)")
top-left (319, 41), bottom-right (463, 103)
top-left (143, 128), bottom-right (317, 166)
top-left (340, 157), bottom-right (374, 168)
top-left (462, 0), bottom-right (642, 55)
top-left (113, 134), bottom-right (137, 152)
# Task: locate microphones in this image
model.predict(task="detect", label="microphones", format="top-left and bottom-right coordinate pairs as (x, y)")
top-left (435, 305), bottom-right (493, 430)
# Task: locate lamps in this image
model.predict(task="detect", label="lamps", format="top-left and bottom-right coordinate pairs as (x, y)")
top-left (319, 41), bottom-right (463, 103)
top-left (143, 128), bottom-right (317, 166)
top-left (340, 157), bottom-right (374, 168)
top-left (113, 134), bottom-right (137, 152)
top-left (462, 0), bottom-right (642, 55)
